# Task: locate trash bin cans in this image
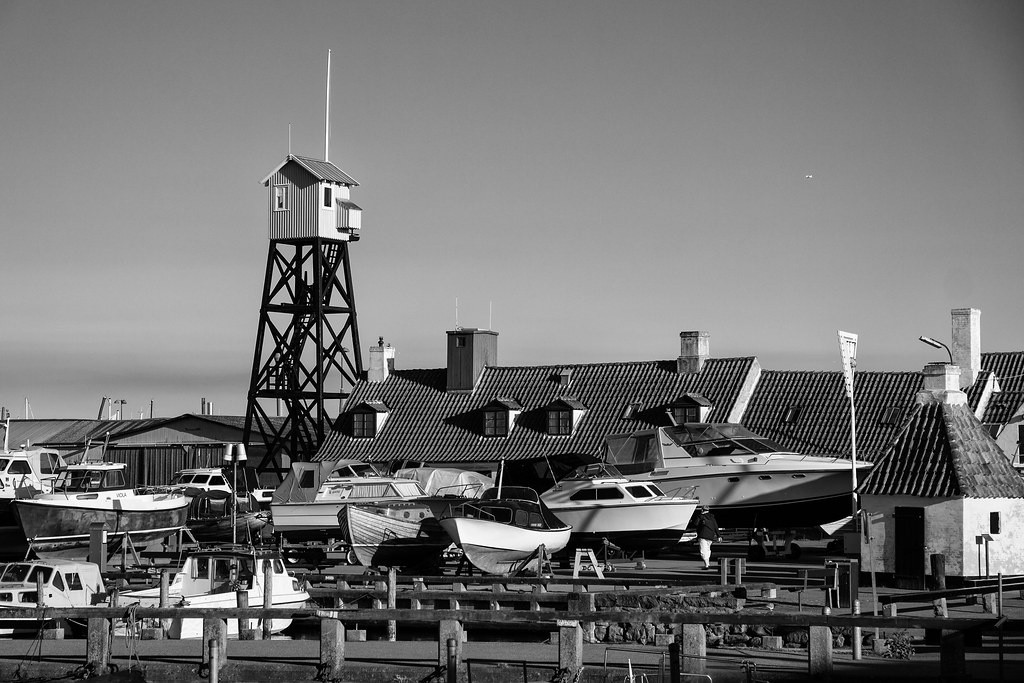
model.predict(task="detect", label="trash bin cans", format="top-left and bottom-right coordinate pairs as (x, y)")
top-left (821, 556), bottom-right (860, 610)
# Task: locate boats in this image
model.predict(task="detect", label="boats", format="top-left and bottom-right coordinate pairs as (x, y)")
top-left (0, 439), bottom-right (73, 533)
top-left (97, 546), bottom-right (310, 642)
top-left (333, 484), bottom-right (478, 572)
top-left (539, 473), bottom-right (702, 557)
top-left (573, 418), bottom-right (874, 512)
top-left (136, 464), bottom-right (276, 516)
top-left (268, 458), bottom-right (492, 528)
top-left (437, 481), bottom-right (572, 578)
top-left (14, 462), bottom-right (193, 555)
top-left (0, 559), bottom-right (108, 638)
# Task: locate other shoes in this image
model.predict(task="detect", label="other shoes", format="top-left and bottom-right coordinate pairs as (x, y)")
top-left (702, 567), bottom-right (709, 570)
